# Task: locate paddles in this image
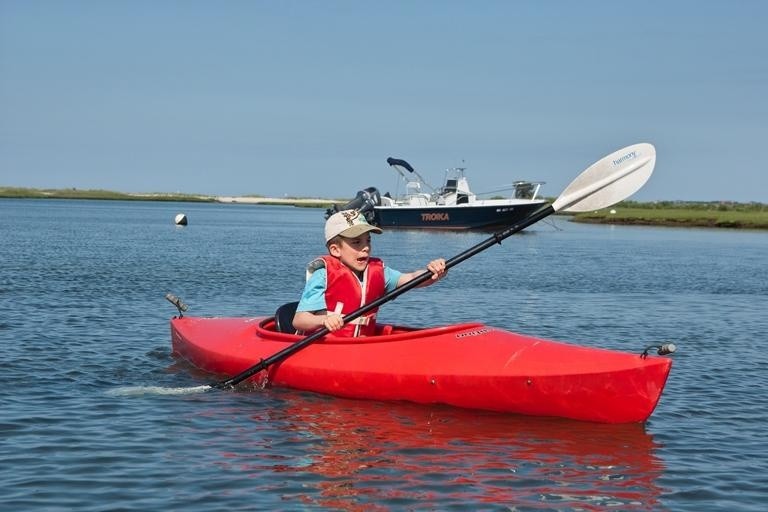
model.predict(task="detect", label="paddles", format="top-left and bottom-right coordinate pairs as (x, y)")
top-left (109, 143), bottom-right (656, 393)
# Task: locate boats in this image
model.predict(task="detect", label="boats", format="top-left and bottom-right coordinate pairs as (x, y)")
top-left (325, 157), bottom-right (549, 233)
top-left (162, 291), bottom-right (673, 427)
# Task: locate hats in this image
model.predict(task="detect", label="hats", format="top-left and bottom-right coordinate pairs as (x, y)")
top-left (324, 209), bottom-right (383, 247)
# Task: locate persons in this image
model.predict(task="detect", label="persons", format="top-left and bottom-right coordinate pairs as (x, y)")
top-left (289, 210), bottom-right (450, 337)
